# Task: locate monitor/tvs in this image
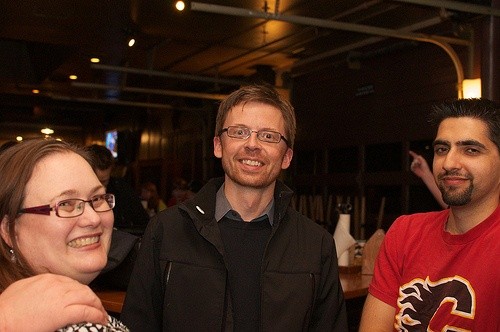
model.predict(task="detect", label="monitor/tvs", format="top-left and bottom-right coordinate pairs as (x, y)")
top-left (105, 130), bottom-right (119, 152)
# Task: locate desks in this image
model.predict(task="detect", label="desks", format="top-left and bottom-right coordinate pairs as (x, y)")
top-left (337, 271), bottom-right (372, 301)
top-left (95, 289), bottom-right (125, 313)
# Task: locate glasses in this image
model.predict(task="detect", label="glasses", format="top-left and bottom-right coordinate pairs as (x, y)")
top-left (218, 126), bottom-right (290, 147)
top-left (18, 194), bottom-right (115, 218)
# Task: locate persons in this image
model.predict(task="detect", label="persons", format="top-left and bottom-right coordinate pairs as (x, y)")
top-left (75, 129), bottom-right (198, 239)
top-left (408, 150), bottom-right (448, 210)
top-left (359, 97), bottom-right (500, 332)
top-left (120, 81), bottom-right (347, 332)
top-left (0, 273), bottom-right (110, 332)
top-left (0, 137), bottom-right (129, 332)
